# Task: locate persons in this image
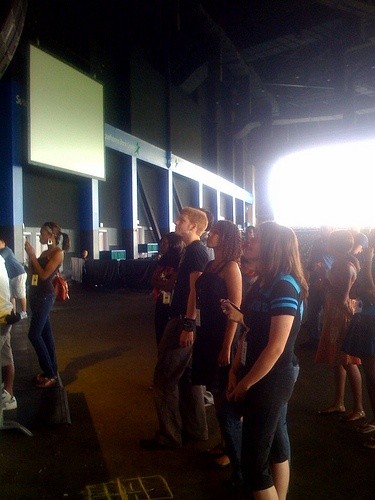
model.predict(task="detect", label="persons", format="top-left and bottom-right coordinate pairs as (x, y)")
top-left (152, 207), bottom-right (375, 500)
top-left (0, 237), bottom-right (28, 320)
top-left (80, 249), bottom-right (91, 261)
top-left (0, 254), bottom-right (16, 410)
top-left (25, 222), bottom-right (69, 387)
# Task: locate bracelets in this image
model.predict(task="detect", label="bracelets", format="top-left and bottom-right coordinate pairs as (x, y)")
top-left (183, 317), bottom-right (195, 332)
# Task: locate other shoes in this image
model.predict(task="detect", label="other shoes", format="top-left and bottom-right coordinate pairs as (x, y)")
top-left (140, 430), bottom-right (164, 447)
top-left (211, 452), bottom-right (232, 469)
top-left (360, 433), bottom-right (375, 450)
top-left (36, 377), bottom-right (56, 389)
top-left (352, 417), bottom-right (375, 434)
top-left (201, 442), bottom-right (226, 458)
top-left (316, 400), bottom-right (347, 416)
top-left (338, 408), bottom-right (366, 423)
top-left (34, 372), bottom-right (57, 383)
top-left (181, 429), bottom-right (207, 441)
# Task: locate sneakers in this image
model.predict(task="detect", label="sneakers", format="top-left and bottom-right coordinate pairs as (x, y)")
top-left (1, 389), bottom-right (17, 410)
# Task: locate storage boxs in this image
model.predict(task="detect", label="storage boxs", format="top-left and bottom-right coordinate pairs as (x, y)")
top-left (99, 250), bottom-right (127, 261)
top-left (138, 243), bottom-right (159, 253)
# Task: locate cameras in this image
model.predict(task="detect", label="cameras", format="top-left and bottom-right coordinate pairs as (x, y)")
top-left (222, 306), bottom-right (228, 312)
top-left (25, 235), bottom-right (31, 243)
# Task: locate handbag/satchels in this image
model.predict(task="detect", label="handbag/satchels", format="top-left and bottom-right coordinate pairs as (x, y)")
top-left (52, 274), bottom-right (70, 303)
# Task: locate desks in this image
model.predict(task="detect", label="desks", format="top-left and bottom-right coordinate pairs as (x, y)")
top-left (82, 257), bottom-right (158, 289)
